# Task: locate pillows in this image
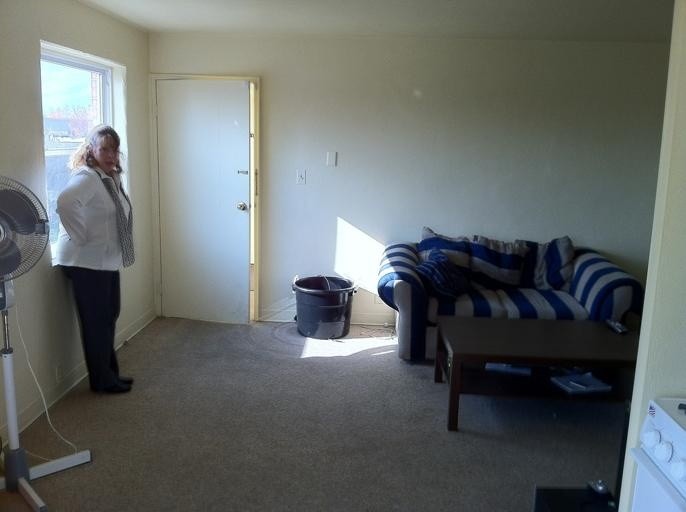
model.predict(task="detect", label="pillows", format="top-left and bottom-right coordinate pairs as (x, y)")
top-left (416, 226), bottom-right (575, 302)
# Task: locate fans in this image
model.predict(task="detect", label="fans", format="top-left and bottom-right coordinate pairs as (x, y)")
top-left (0, 174), bottom-right (92, 512)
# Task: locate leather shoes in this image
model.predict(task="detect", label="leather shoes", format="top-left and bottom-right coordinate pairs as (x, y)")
top-left (90, 376), bottom-right (133, 394)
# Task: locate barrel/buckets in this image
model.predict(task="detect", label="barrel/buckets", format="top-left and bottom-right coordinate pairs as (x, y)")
top-left (292, 271), bottom-right (358, 340)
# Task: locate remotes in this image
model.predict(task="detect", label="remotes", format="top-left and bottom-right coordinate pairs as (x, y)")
top-left (606, 320), bottom-right (629, 334)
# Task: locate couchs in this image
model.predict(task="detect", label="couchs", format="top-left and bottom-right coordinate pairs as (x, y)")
top-left (378, 243), bottom-right (643, 365)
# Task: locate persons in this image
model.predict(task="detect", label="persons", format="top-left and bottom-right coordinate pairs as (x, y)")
top-left (57, 123), bottom-right (134, 393)
